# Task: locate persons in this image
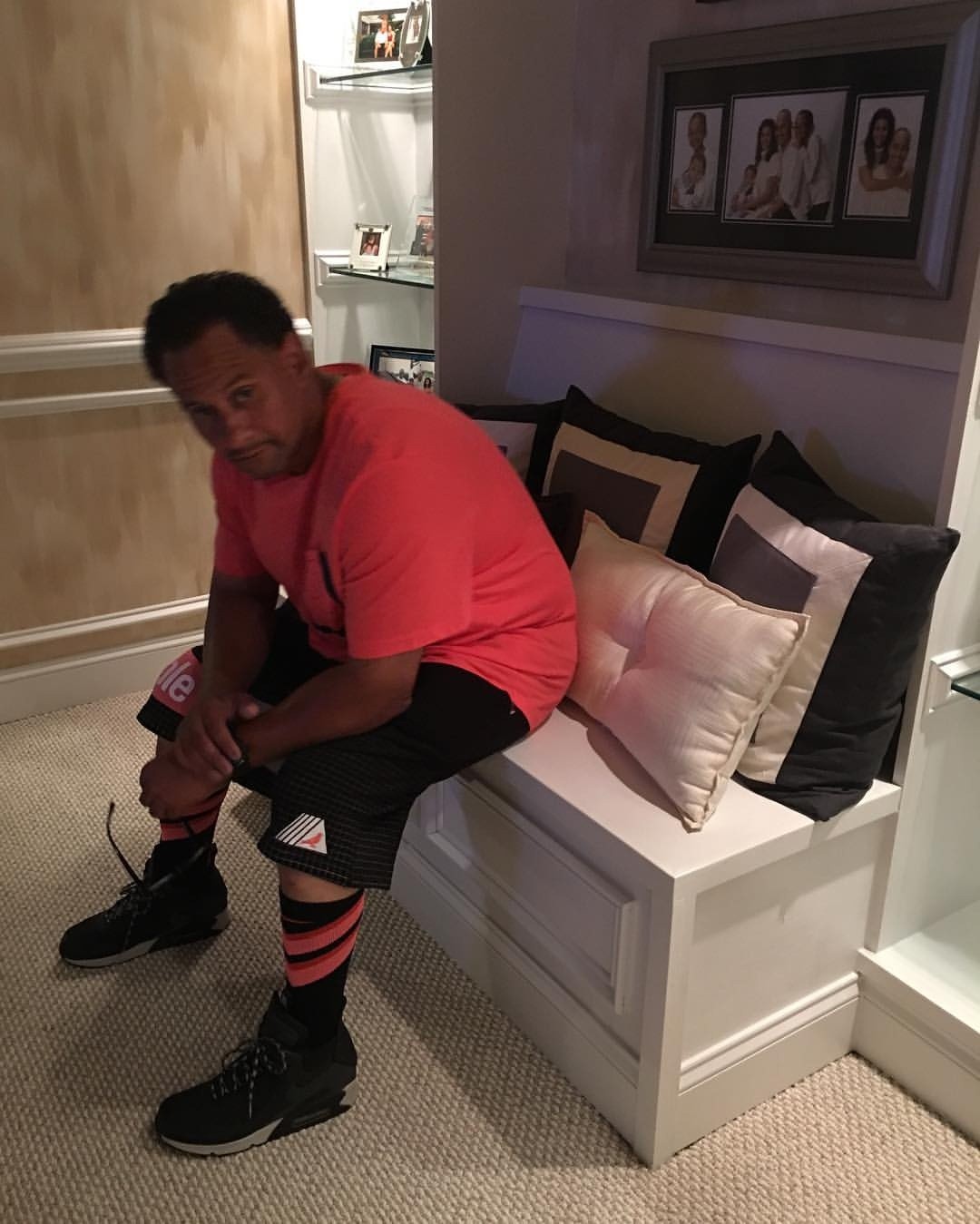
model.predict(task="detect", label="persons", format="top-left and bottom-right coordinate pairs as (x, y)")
top-left (374, 21), bottom-right (395, 58)
top-left (725, 108), bottom-right (834, 221)
top-left (410, 218), bottom-right (436, 256)
top-left (671, 112), bottom-right (718, 211)
top-left (424, 378), bottom-right (432, 392)
top-left (59, 271), bottom-right (579, 1157)
top-left (395, 369), bottom-right (414, 387)
top-left (361, 233), bottom-right (379, 255)
top-left (846, 107), bottom-right (916, 217)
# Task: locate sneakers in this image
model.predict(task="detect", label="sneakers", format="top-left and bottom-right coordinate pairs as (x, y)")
top-left (57, 801), bottom-right (229, 966)
top-left (158, 991), bottom-right (356, 1154)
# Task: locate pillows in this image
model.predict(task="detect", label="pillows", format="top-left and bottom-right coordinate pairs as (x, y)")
top-left (706, 429), bottom-right (964, 824)
top-left (560, 509), bottom-right (812, 830)
top-left (448, 398), bottom-right (566, 500)
top-left (539, 386), bottom-right (765, 576)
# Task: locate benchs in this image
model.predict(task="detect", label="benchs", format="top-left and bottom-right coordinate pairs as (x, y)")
top-left (274, 584), bottom-right (902, 1172)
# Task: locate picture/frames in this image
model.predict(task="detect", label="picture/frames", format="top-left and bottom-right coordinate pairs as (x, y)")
top-left (406, 211), bottom-right (434, 262)
top-left (636, 0), bottom-right (980, 300)
top-left (398, 0), bottom-right (430, 67)
top-left (368, 343), bottom-right (435, 391)
top-left (355, 8), bottom-right (408, 61)
top-left (348, 222), bottom-right (391, 272)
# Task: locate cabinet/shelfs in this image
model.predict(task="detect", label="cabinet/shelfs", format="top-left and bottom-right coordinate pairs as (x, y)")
top-left (304, 58), bottom-right (434, 293)
top-left (863, 664), bottom-right (980, 1151)
